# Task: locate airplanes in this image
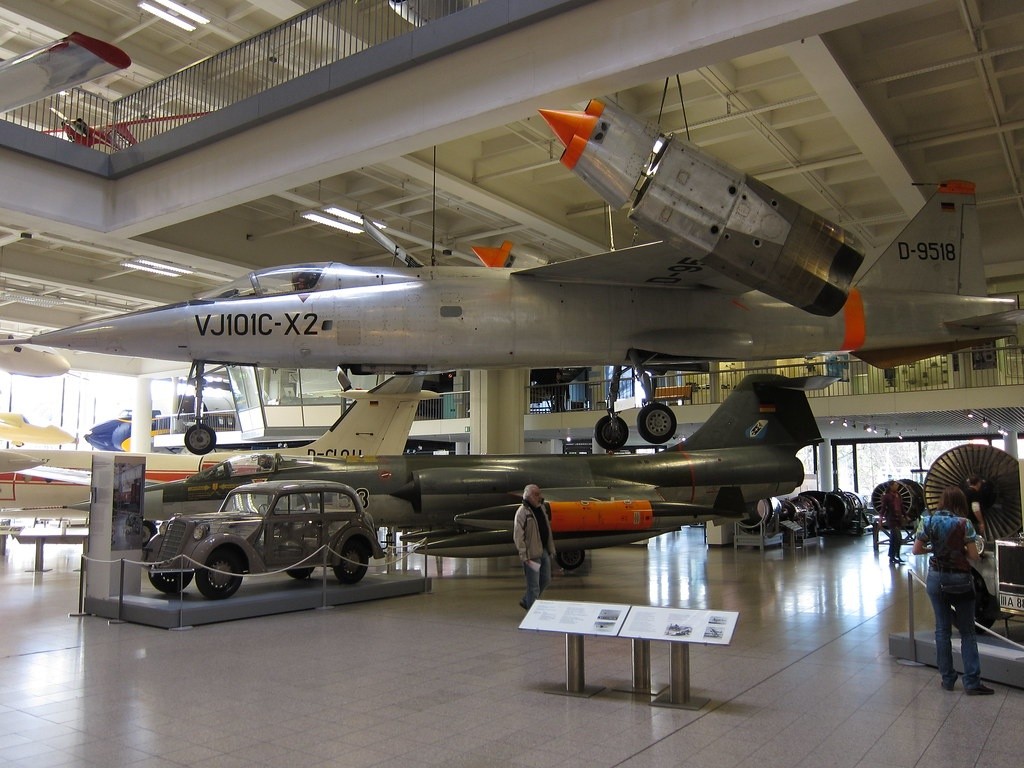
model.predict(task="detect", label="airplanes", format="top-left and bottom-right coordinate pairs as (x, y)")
top-left (0, 413), bottom-right (79, 450)
top-left (21, 374), bottom-right (824, 569)
top-left (0, 374), bottom-right (425, 520)
top-left (0, 100), bottom-right (1024, 455)
top-left (40, 106), bottom-right (215, 156)
top-left (0, 334), bottom-right (71, 377)
top-left (0, 31), bottom-right (131, 116)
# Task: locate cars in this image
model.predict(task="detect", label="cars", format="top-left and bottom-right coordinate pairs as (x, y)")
top-left (142, 480), bottom-right (386, 600)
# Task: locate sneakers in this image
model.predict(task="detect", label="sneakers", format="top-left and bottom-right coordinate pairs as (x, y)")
top-left (966, 683), bottom-right (995, 695)
top-left (941, 682), bottom-right (953, 690)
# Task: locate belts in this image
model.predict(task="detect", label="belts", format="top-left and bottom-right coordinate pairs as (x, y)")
top-left (933, 565), bottom-right (967, 574)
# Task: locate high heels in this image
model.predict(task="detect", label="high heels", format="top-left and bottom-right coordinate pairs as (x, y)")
top-left (889, 556), bottom-right (905, 563)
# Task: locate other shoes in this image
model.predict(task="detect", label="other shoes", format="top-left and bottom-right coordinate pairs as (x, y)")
top-left (519, 599), bottom-right (528, 609)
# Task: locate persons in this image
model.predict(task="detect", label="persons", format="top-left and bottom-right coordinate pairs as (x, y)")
top-left (912, 487), bottom-right (994, 696)
top-left (513, 484), bottom-right (556, 611)
top-left (551, 371), bottom-right (571, 411)
top-left (879, 480), bottom-right (911, 563)
